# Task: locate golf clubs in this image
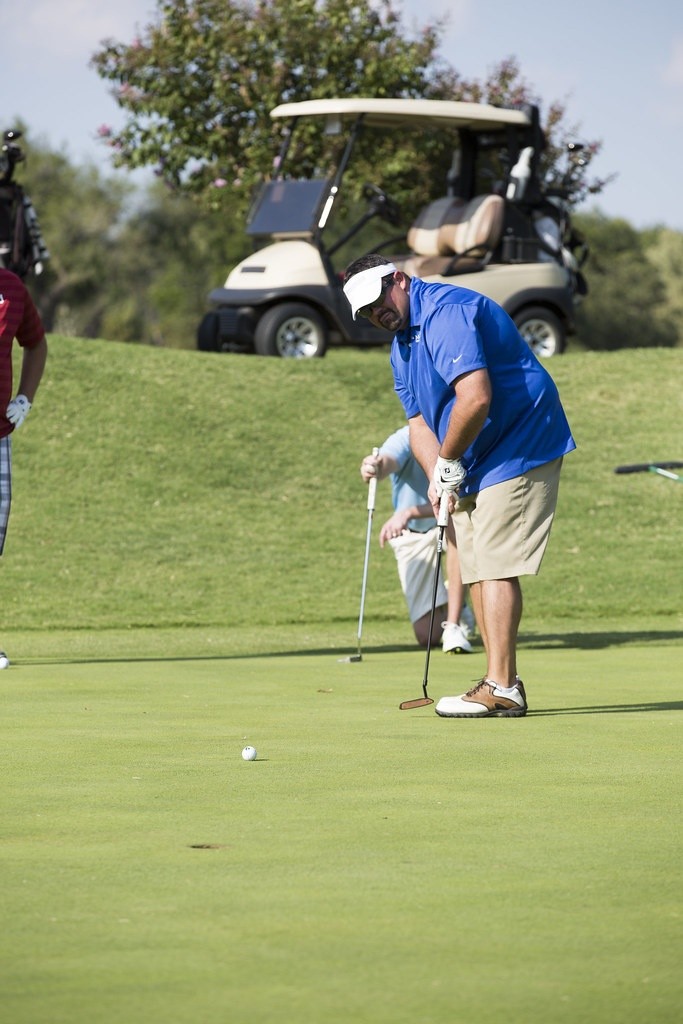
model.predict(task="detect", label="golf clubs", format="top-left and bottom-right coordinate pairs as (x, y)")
top-left (399, 488), bottom-right (454, 711)
top-left (346, 446), bottom-right (380, 663)
top-left (561, 141), bottom-right (588, 189)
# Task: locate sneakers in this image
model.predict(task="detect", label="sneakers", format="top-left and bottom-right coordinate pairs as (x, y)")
top-left (435, 675), bottom-right (528, 718)
top-left (441, 621), bottom-right (474, 653)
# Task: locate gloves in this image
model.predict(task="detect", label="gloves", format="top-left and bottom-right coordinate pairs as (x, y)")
top-left (5, 394), bottom-right (32, 429)
top-left (433, 456), bottom-right (467, 497)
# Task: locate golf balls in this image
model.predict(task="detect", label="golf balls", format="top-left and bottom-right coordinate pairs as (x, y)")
top-left (241, 746), bottom-right (257, 761)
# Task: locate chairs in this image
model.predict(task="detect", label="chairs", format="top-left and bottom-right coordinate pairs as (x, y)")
top-left (387, 193), bottom-right (511, 278)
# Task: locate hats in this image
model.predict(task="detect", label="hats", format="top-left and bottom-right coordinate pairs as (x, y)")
top-left (343, 263), bottom-right (397, 322)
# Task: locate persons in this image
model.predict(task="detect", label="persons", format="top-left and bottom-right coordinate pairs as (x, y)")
top-left (361, 425), bottom-right (473, 654)
top-left (343, 254), bottom-right (577, 716)
top-left (0, 258), bottom-right (48, 668)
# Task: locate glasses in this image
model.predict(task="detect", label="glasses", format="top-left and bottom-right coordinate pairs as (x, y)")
top-left (357, 275), bottom-right (393, 318)
